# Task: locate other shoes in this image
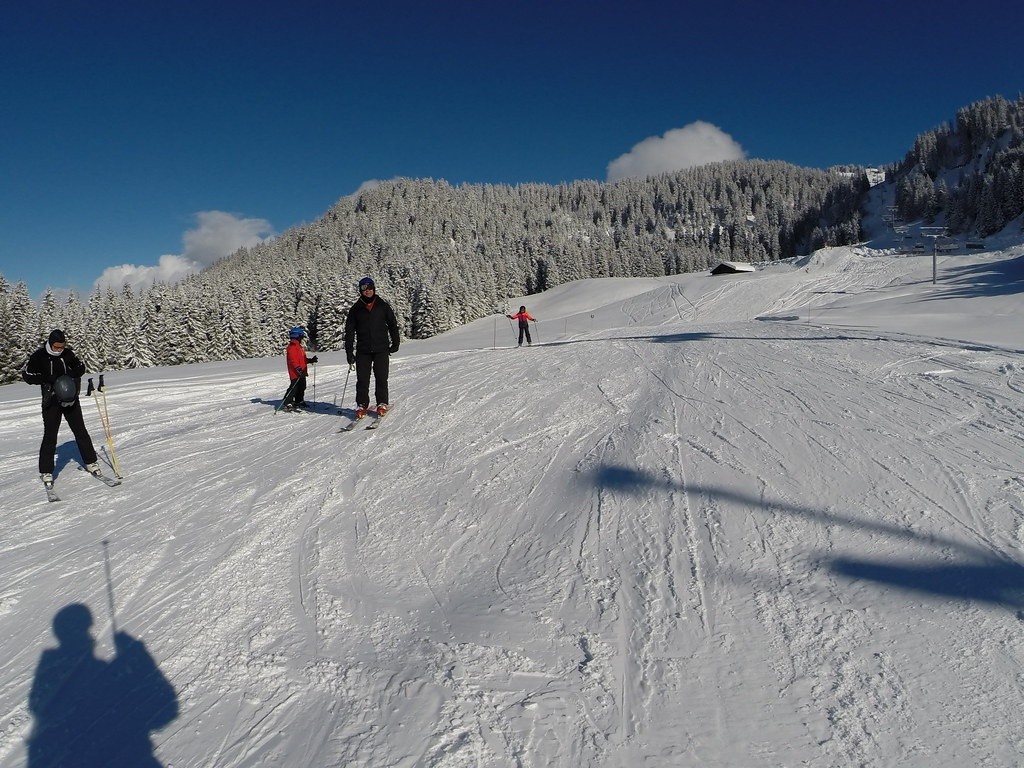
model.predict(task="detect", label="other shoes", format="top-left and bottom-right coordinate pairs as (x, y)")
top-left (377, 405), bottom-right (385, 416)
top-left (294, 401), bottom-right (310, 409)
top-left (87, 462), bottom-right (101, 474)
top-left (355, 406), bottom-right (366, 419)
top-left (282, 396), bottom-right (294, 408)
top-left (42, 472), bottom-right (54, 486)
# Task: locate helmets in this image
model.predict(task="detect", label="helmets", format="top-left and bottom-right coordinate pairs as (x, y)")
top-left (289, 326), bottom-right (305, 341)
top-left (54, 375), bottom-right (76, 402)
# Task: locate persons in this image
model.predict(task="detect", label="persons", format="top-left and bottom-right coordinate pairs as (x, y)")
top-left (506, 305), bottom-right (536, 346)
top-left (282, 327), bottom-right (318, 407)
top-left (345, 277), bottom-right (401, 418)
top-left (22, 328), bottom-right (105, 489)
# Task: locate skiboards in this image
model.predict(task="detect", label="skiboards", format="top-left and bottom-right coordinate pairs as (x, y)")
top-left (39, 465), bottom-right (123, 502)
top-left (339, 402), bottom-right (395, 431)
top-left (282, 400), bottom-right (332, 415)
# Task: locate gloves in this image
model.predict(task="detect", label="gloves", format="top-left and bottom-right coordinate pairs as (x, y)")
top-left (347, 352), bottom-right (356, 365)
top-left (44, 374), bottom-right (58, 386)
top-left (389, 342), bottom-right (399, 353)
top-left (65, 373), bottom-right (72, 378)
top-left (295, 367), bottom-right (305, 379)
top-left (309, 355), bottom-right (318, 363)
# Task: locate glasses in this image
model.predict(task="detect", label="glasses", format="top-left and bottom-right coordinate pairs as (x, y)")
top-left (299, 333), bottom-right (304, 339)
top-left (360, 285), bottom-right (374, 291)
top-left (53, 344), bottom-right (65, 349)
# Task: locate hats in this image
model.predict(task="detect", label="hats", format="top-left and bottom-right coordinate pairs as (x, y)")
top-left (359, 277), bottom-right (374, 291)
